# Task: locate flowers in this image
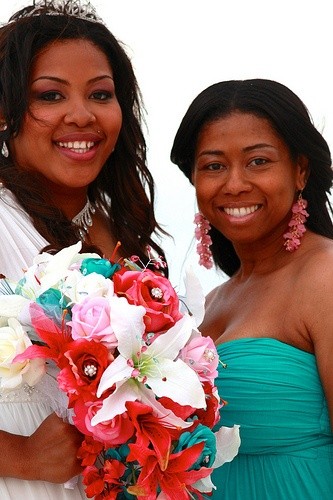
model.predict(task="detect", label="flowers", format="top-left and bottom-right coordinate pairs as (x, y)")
top-left (0, 240), bottom-right (242, 500)
top-left (194, 210), bottom-right (214, 269)
top-left (283, 195), bottom-right (310, 252)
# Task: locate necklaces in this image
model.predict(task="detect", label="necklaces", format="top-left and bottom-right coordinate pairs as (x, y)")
top-left (71, 195), bottom-right (96, 244)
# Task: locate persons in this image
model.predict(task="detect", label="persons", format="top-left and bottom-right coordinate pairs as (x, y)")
top-left (169, 78), bottom-right (333, 500)
top-left (0, 0), bottom-right (168, 500)
